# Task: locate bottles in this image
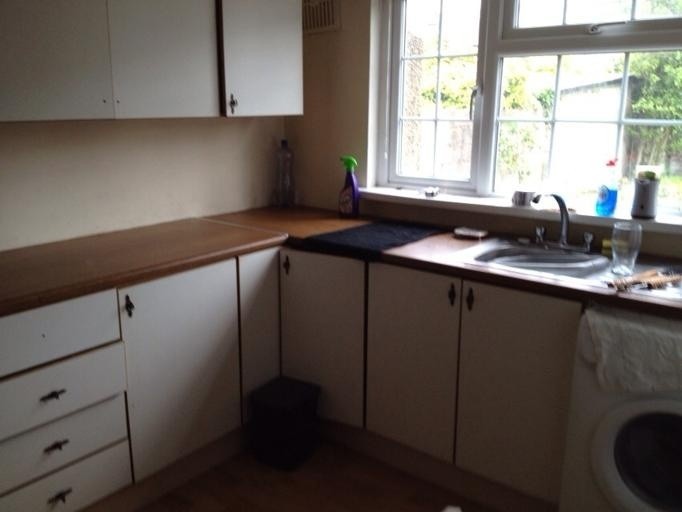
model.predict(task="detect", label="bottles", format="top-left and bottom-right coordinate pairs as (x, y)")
top-left (274, 140), bottom-right (294, 209)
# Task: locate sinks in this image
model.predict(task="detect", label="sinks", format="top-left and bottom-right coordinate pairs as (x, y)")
top-left (464, 241), bottom-right (608, 282)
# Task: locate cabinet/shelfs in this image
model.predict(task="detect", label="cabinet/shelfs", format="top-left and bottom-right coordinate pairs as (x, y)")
top-left (1, 286), bottom-right (132, 509)
top-left (366, 260), bottom-right (583, 508)
top-left (278, 244), bottom-right (365, 433)
top-left (219, 0), bottom-right (304, 117)
top-left (0, 0), bottom-right (220, 122)
top-left (117, 256), bottom-right (242, 483)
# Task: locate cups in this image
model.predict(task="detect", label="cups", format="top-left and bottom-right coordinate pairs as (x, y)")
top-left (611, 222), bottom-right (642, 276)
top-left (630, 165), bottom-right (662, 219)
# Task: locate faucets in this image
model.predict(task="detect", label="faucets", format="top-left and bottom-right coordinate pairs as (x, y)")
top-left (512, 190), bottom-right (570, 245)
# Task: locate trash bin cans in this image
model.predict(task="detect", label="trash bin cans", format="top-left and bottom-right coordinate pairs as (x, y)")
top-left (248, 374), bottom-right (321, 470)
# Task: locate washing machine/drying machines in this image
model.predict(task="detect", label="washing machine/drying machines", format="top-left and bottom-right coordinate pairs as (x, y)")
top-left (559, 308), bottom-right (682, 512)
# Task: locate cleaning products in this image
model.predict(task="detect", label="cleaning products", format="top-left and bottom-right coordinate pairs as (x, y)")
top-left (594, 159), bottom-right (620, 218)
top-left (338, 156), bottom-right (360, 217)
